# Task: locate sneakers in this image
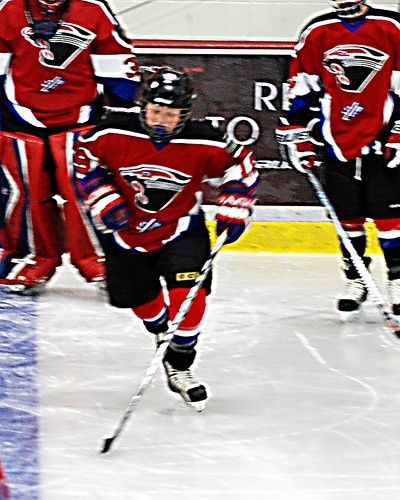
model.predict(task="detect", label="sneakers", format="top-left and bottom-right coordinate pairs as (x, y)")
top-left (162, 358), bottom-right (208, 412)
top-left (337, 256), bottom-right (372, 322)
top-left (387, 266), bottom-right (400, 322)
top-left (156, 331), bottom-right (167, 349)
top-left (78, 255), bottom-right (107, 291)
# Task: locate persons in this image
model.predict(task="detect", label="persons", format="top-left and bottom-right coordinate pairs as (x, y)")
top-left (275, 0), bottom-right (400, 322)
top-left (0, 1), bottom-right (143, 287)
top-left (73, 65), bottom-right (261, 402)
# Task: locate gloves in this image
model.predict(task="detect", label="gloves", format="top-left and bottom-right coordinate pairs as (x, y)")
top-left (384, 119), bottom-right (400, 168)
top-left (275, 115), bottom-right (317, 174)
top-left (84, 183), bottom-right (130, 234)
top-left (215, 193), bottom-right (257, 245)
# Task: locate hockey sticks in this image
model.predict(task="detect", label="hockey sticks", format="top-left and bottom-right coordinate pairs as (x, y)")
top-left (97, 228), bottom-right (228, 453)
top-left (304, 164), bottom-right (400, 339)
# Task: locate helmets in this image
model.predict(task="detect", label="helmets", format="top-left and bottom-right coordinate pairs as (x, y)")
top-left (134, 67), bottom-right (198, 144)
